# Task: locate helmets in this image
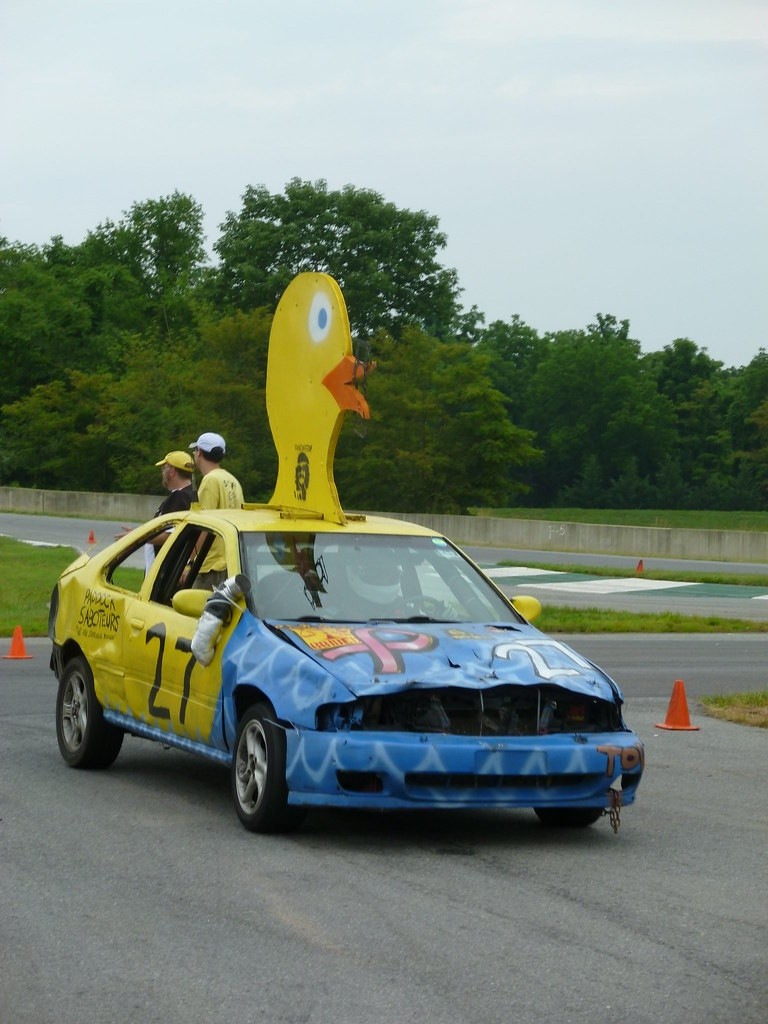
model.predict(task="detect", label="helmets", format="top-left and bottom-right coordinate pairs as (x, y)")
top-left (347, 546), bottom-right (403, 605)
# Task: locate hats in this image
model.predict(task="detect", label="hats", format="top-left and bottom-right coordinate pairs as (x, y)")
top-left (189, 432), bottom-right (225, 455)
top-left (155, 451), bottom-right (194, 472)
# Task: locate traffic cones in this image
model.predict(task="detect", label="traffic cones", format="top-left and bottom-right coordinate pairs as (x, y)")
top-left (3, 626), bottom-right (32, 660)
top-left (655, 680), bottom-right (701, 730)
top-left (86, 530), bottom-right (97, 543)
top-left (636, 559), bottom-right (644, 574)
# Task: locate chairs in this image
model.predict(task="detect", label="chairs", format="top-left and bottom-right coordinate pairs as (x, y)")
top-left (258, 572), bottom-right (307, 618)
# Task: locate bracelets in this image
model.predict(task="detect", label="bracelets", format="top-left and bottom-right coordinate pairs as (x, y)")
top-left (187, 560), bottom-right (193, 565)
top-left (184, 565), bottom-right (191, 571)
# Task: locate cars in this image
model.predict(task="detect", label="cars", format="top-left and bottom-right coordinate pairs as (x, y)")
top-left (50, 506), bottom-right (643, 837)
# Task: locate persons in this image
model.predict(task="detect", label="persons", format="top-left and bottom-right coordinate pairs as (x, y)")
top-left (179, 432), bottom-right (246, 590)
top-left (342, 541), bottom-right (459, 621)
top-left (115, 451), bottom-right (198, 581)
